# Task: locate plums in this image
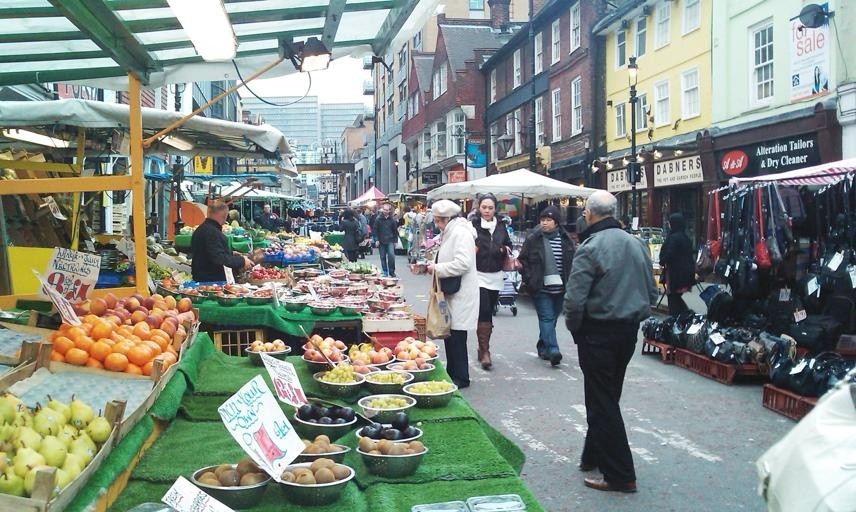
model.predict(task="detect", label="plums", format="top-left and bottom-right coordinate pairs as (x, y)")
top-left (297, 402), bottom-right (355, 424)
top-left (360, 412), bottom-right (417, 440)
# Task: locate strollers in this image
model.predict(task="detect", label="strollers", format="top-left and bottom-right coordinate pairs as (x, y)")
top-left (362, 224), bottom-right (373, 256)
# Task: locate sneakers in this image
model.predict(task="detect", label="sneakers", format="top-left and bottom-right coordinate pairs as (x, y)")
top-left (537, 340), bottom-right (562, 366)
top-left (383, 270), bottom-right (396, 277)
top-left (450, 378), bottom-right (469, 390)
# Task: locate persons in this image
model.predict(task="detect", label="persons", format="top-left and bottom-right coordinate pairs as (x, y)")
top-left (469, 193), bottom-right (513, 368)
top-left (517, 205), bottom-right (575, 365)
top-left (191, 201), bottom-right (404, 281)
top-left (564, 189), bottom-right (658, 492)
top-left (577, 208), bottom-right (589, 244)
top-left (411, 200), bottom-right (480, 390)
top-left (660, 213), bottom-right (696, 316)
top-left (619, 216), bottom-right (633, 234)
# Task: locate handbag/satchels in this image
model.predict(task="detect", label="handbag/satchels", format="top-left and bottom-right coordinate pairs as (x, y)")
top-left (356, 228), bottom-right (364, 243)
top-left (503, 245), bottom-right (523, 270)
top-left (645, 222), bottom-right (856, 398)
top-left (424, 288), bottom-right (452, 340)
top-left (757, 378), bottom-right (856, 511)
top-left (435, 275), bottom-right (461, 294)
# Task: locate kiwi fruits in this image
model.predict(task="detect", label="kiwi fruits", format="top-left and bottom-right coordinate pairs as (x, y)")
top-left (360, 436), bottom-right (426, 454)
top-left (196, 460), bottom-right (268, 487)
top-left (280, 435), bottom-right (351, 485)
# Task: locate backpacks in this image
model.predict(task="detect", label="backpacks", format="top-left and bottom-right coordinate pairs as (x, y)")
top-left (774, 187), bottom-right (806, 221)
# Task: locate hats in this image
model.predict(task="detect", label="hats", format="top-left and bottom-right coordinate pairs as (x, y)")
top-left (539, 205), bottom-right (561, 223)
top-left (432, 199), bottom-right (462, 217)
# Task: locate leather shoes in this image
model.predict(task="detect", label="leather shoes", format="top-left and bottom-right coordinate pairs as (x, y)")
top-left (579, 459), bottom-right (597, 470)
top-left (584, 474), bottom-right (638, 493)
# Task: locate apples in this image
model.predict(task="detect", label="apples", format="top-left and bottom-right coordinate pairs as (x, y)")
top-left (70, 292), bottom-right (196, 343)
top-left (251, 339), bottom-right (286, 352)
top-left (304, 334), bottom-right (437, 374)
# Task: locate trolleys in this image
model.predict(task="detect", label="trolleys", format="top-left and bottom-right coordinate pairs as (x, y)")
top-left (495, 276), bottom-right (520, 315)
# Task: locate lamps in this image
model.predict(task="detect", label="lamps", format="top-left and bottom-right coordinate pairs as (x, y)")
top-left (164, 0), bottom-right (240, 62)
top-left (588, 137), bottom-right (687, 176)
top-left (277, 31), bottom-right (331, 74)
top-left (494, 114), bottom-right (527, 155)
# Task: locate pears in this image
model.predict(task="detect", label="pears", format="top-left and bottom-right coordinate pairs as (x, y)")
top-left (0, 392), bottom-right (112, 500)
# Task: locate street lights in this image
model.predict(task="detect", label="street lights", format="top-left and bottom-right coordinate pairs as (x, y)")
top-left (407, 160), bottom-right (418, 193)
top-left (626, 54), bottom-right (643, 232)
top-left (498, 115), bottom-right (537, 173)
top-left (463, 127), bottom-right (470, 181)
top-left (392, 159), bottom-right (399, 191)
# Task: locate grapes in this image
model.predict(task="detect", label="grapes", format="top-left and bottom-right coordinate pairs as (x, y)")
top-left (318, 366), bottom-right (453, 408)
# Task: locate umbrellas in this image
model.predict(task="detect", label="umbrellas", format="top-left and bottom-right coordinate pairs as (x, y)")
top-left (456, 168), bottom-right (598, 217)
top-left (427, 181), bottom-right (511, 201)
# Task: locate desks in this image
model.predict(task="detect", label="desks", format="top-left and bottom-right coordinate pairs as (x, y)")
top-left (0, 212), bottom-right (544, 512)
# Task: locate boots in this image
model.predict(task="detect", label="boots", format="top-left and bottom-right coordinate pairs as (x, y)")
top-left (476, 320), bottom-right (492, 367)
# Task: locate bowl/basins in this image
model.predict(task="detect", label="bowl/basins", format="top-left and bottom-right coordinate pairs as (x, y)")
top-left (301, 344), bottom-right (439, 367)
top-left (386, 361), bottom-right (436, 382)
top-left (276, 463), bottom-right (357, 505)
top-left (244, 346), bottom-right (292, 366)
top-left (312, 372), bottom-right (365, 396)
top-left (190, 463), bottom-right (268, 506)
top-left (405, 380), bottom-right (459, 407)
top-left (300, 443), bottom-right (352, 465)
top-left (300, 354), bottom-right (349, 373)
top-left (361, 446), bottom-right (429, 476)
top-left (294, 411), bottom-right (359, 440)
top-left (158, 268), bottom-right (402, 315)
top-left (358, 395), bottom-right (418, 418)
top-left (358, 425), bottom-right (423, 442)
top-left (366, 373), bottom-right (415, 394)
